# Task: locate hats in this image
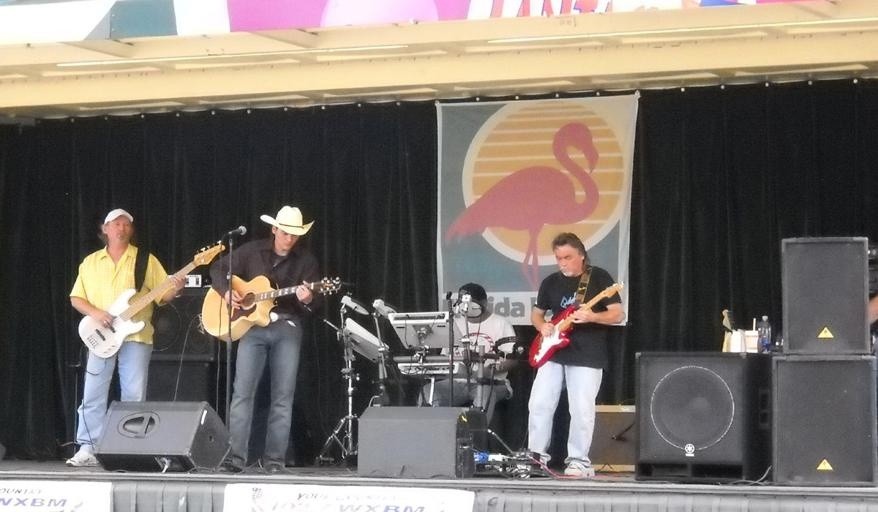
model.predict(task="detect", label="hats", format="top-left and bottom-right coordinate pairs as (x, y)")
top-left (260, 206), bottom-right (315, 236)
top-left (453, 282), bottom-right (486, 306)
top-left (103, 208), bottom-right (133, 224)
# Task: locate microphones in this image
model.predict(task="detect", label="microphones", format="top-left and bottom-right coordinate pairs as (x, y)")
top-left (446, 289), bottom-right (454, 301)
top-left (224, 224), bottom-right (248, 246)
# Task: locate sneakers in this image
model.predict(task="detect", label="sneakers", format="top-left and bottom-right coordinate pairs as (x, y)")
top-left (65, 447), bottom-right (101, 466)
top-left (564, 459), bottom-right (595, 477)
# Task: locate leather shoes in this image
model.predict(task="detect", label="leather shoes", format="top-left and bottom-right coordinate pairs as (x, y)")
top-left (263, 457), bottom-right (281, 472)
top-left (225, 462), bottom-right (242, 472)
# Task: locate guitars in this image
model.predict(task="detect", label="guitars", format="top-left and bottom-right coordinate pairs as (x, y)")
top-left (202, 274), bottom-right (342, 342)
top-left (529, 282), bottom-right (625, 368)
top-left (78, 242), bottom-right (225, 358)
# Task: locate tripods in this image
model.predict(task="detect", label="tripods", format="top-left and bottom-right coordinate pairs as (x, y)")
top-left (322, 380), bottom-right (358, 469)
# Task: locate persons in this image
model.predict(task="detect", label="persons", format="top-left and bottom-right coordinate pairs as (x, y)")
top-left (526, 234), bottom-right (626, 479)
top-left (416, 282), bottom-right (520, 426)
top-left (208, 205), bottom-right (323, 473)
top-left (65, 206), bottom-right (187, 466)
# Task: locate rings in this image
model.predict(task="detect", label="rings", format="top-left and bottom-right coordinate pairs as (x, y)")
top-left (103, 320), bottom-right (107, 324)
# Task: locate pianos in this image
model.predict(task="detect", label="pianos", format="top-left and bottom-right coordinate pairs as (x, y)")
top-left (390, 355), bottom-right (480, 374)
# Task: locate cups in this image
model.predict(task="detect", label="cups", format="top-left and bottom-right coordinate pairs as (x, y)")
top-left (745, 330), bottom-right (758, 353)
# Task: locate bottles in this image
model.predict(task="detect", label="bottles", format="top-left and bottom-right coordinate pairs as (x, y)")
top-left (731, 332), bottom-right (742, 353)
top-left (758, 316), bottom-right (772, 353)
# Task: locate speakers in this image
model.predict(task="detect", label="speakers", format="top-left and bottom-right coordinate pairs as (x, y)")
top-left (93, 399), bottom-right (231, 473)
top-left (633, 349), bottom-right (752, 485)
top-left (781, 236), bottom-right (869, 355)
top-left (358, 407), bottom-right (484, 479)
top-left (770, 353), bottom-right (876, 487)
